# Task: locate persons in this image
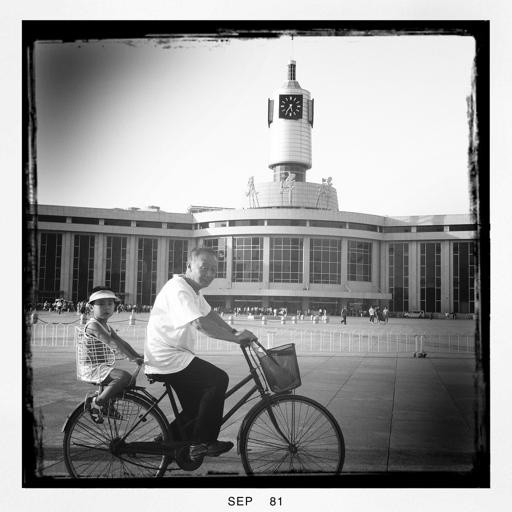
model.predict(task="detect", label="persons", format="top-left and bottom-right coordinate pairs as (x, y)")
top-left (340, 305), bottom-right (390, 325)
top-left (76, 286), bottom-right (146, 425)
top-left (42, 298), bottom-right (150, 316)
top-left (142, 246), bottom-right (258, 461)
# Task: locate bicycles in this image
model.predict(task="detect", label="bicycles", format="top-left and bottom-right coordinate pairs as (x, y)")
top-left (59, 334), bottom-right (348, 480)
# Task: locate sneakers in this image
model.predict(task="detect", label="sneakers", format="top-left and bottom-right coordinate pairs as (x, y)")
top-left (189, 440), bottom-right (233, 461)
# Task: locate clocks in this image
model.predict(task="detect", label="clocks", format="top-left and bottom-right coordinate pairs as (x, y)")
top-left (278, 95), bottom-right (303, 120)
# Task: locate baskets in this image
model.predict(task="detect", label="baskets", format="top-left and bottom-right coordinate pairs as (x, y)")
top-left (256, 343), bottom-right (301, 393)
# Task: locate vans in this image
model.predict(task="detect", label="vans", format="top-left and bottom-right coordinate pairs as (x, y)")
top-left (404, 309), bottom-right (425, 319)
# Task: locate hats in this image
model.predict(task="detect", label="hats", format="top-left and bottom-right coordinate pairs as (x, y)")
top-left (88, 289), bottom-right (122, 303)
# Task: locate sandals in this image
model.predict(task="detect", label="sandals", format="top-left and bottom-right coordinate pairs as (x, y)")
top-left (90, 396), bottom-right (104, 423)
